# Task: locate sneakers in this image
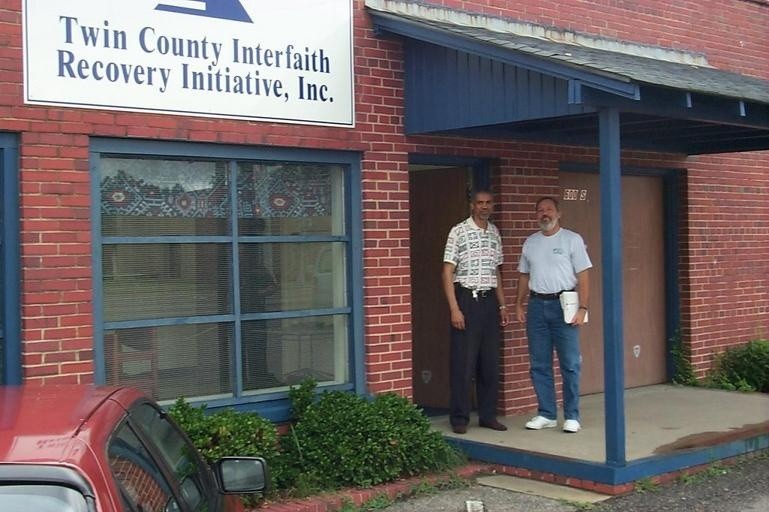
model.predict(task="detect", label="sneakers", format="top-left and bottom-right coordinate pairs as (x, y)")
top-left (524, 414), bottom-right (558, 430)
top-left (562, 418), bottom-right (581, 433)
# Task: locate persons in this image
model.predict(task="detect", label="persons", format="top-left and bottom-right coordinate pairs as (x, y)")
top-left (514, 196), bottom-right (594, 433)
top-left (441, 188), bottom-right (511, 435)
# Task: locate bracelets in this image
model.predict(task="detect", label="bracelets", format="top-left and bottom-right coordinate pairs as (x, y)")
top-left (578, 305), bottom-right (588, 311)
top-left (499, 304), bottom-right (508, 311)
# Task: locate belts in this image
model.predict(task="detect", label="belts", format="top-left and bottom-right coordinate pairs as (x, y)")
top-left (529, 289), bottom-right (576, 300)
top-left (457, 284), bottom-right (496, 300)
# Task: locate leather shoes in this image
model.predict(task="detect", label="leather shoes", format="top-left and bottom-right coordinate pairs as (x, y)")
top-left (453, 423), bottom-right (467, 434)
top-left (478, 417), bottom-right (508, 432)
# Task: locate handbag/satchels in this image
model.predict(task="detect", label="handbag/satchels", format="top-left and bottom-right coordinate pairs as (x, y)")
top-left (558, 290), bottom-right (589, 325)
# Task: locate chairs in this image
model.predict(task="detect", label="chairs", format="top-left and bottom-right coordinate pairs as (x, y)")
top-left (104, 327), bottom-right (159, 401)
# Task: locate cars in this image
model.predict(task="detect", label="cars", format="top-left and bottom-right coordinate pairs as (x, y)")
top-left (1, 381), bottom-right (270, 512)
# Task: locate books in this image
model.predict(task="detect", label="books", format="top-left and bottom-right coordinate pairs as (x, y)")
top-left (559, 290), bottom-right (579, 324)
